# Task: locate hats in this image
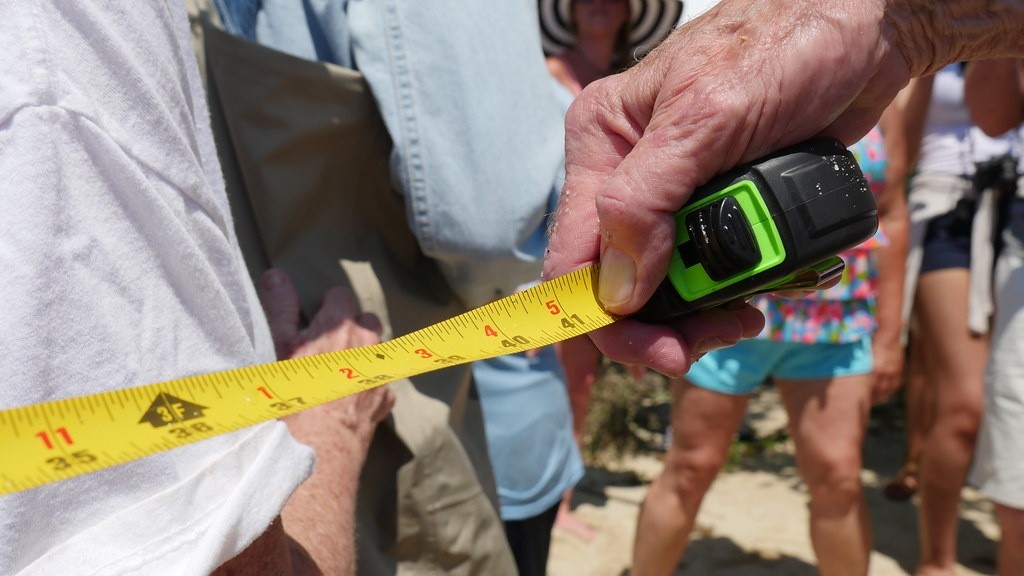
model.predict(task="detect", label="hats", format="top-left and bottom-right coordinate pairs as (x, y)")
top-left (537, 0), bottom-right (683, 74)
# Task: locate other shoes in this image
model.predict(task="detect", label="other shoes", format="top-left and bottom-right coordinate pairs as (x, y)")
top-left (884, 462), bottom-right (919, 501)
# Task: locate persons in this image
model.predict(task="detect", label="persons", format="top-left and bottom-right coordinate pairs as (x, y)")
top-left (0, 0), bottom-right (1024, 576)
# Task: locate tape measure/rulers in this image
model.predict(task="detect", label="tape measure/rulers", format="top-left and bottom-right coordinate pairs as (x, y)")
top-left (0, 138), bottom-right (879, 497)
